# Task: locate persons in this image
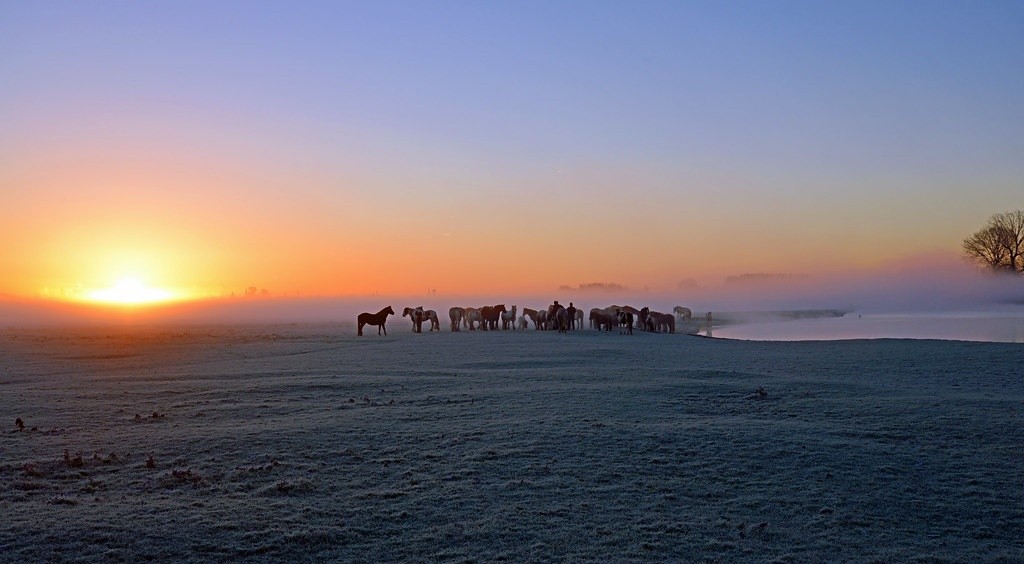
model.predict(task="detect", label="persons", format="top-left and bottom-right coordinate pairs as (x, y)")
top-left (567, 302), bottom-right (576, 330)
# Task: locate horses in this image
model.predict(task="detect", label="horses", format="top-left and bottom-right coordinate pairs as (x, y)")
top-left (449, 304), bottom-right (584, 333)
top-left (357, 306), bottom-right (394, 336)
top-left (589, 305), bottom-right (692, 333)
top-left (403, 306), bottom-right (440, 333)
